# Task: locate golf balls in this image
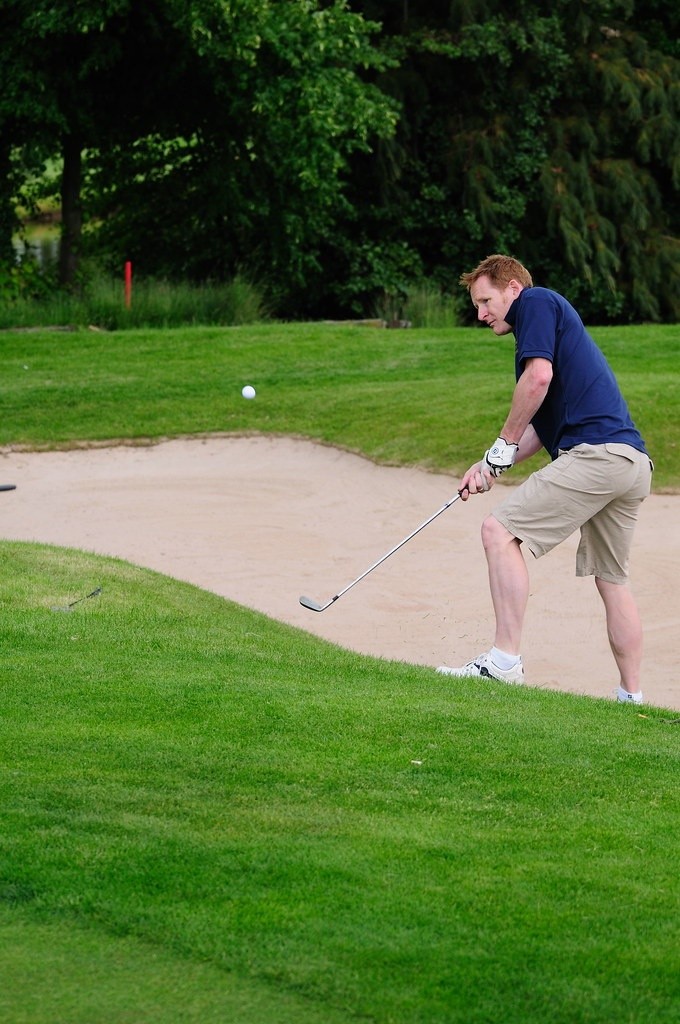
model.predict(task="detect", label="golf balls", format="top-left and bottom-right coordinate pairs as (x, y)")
top-left (242, 385), bottom-right (256, 399)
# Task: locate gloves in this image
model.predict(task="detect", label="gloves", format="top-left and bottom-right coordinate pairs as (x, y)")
top-left (479, 436), bottom-right (519, 491)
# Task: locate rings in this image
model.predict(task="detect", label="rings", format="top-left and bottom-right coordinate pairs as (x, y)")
top-left (479, 488), bottom-right (483, 491)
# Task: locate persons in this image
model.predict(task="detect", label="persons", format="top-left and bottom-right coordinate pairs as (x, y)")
top-left (436, 255), bottom-right (654, 705)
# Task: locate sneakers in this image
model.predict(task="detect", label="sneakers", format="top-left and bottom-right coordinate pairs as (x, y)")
top-left (435, 654), bottom-right (525, 686)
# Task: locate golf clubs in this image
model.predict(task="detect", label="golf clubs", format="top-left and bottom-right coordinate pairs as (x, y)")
top-left (299, 483), bottom-right (470, 612)
